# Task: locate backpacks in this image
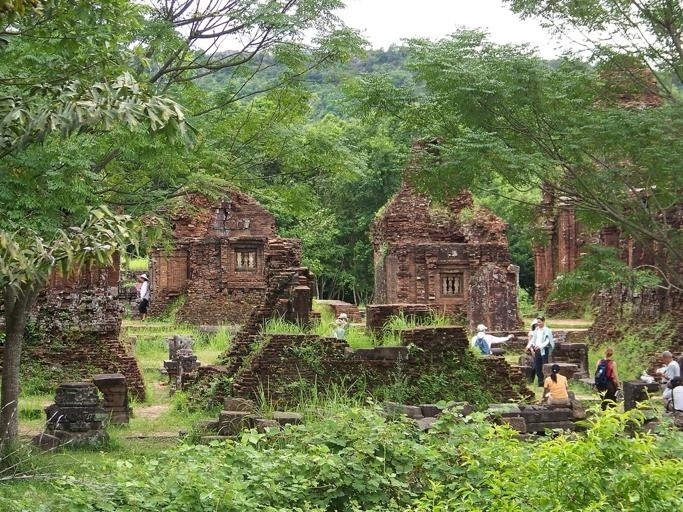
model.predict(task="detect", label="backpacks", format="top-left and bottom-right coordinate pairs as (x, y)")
top-left (475, 336), bottom-right (490, 355)
top-left (595, 359), bottom-right (610, 390)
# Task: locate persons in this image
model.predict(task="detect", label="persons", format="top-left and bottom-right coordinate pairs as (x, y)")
top-left (524, 316), bottom-right (554, 386)
top-left (527, 319), bottom-right (538, 383)
top-left (662, 376), bottom-right (683, 429)
top-left (542, 364), bottom-right (568, 405)
top-left (471, 324), bottom-right (514, 355)
top-left (334, 312), bottom-right (349, 338)
top-left (597, 348), bottom-right (621, 411)
top-left (139, 273), bottom-right (150, 321)
top-left (656, 351), bottom-right (680, 397)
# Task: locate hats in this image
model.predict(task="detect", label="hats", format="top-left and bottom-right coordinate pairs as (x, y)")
top-left (140, 274), bottom-right (150, 281)
top-left (338, 313), bottom-right (347, 319)
top-left (477, 324), bottom-right (487, 332)
